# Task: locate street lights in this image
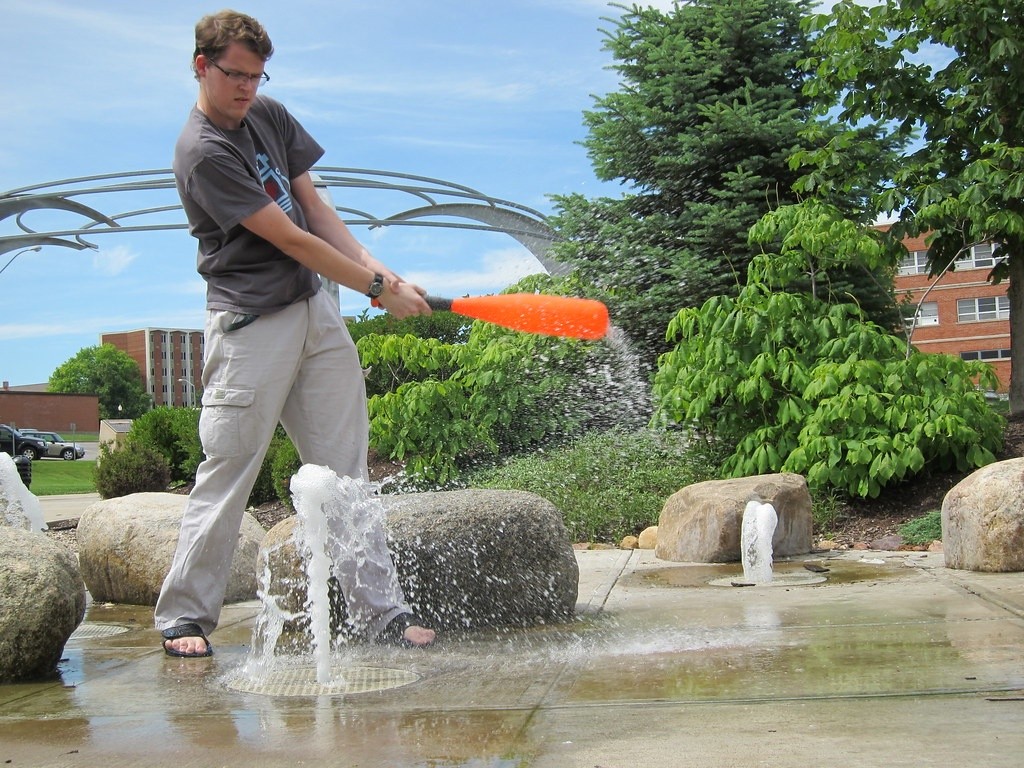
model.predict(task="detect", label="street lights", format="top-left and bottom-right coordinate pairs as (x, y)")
top-left (177, 378), bottom-right (196, 409)
top-left (118, 404), bottom-right (123, 419)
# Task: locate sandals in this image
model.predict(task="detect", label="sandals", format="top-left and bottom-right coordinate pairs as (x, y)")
top-left (162, 623), bottom-right (213, 657)
top-left (377, 611), bottom-right (428, 648)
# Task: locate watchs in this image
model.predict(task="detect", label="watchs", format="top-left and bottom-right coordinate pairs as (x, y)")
top-left (366, 273), bottom-right (384, 298)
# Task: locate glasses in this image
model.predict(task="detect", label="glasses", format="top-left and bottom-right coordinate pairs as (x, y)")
top-left (206, 56), bottom-right (270, 87)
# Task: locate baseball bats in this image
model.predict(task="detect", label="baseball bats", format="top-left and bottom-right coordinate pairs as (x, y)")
top-left (374, 291), bottom-right (612, 341)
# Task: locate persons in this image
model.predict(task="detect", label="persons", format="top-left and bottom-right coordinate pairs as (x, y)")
top-left (155, 11), bottom-right (437, 657)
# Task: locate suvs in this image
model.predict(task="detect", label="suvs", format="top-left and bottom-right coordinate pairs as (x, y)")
top-left (0, 424), bottom-right (50, 462)
top-left (20, 431), bottom-right (85, 460)
